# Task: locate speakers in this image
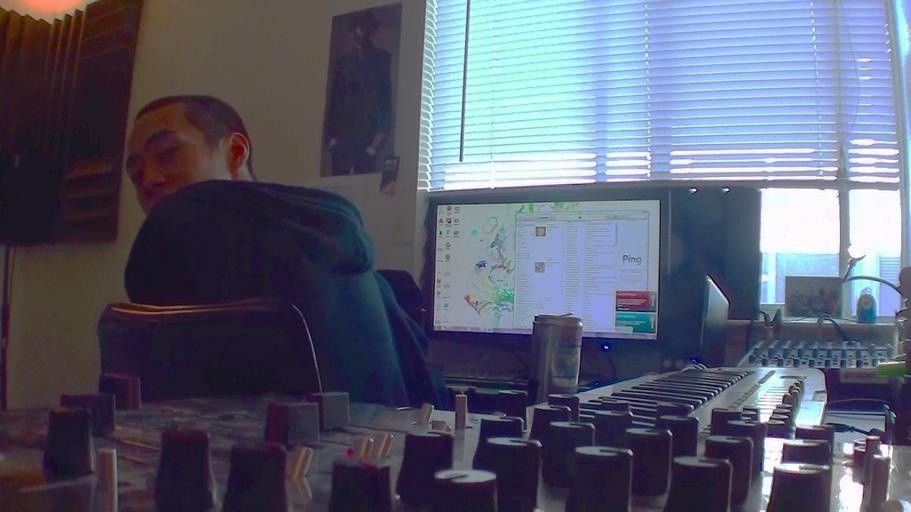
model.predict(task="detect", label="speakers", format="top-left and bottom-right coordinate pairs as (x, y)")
top-left (377, 270), bottom-right (426, 352)
top-left (659, 275), bottom-right (730, 368)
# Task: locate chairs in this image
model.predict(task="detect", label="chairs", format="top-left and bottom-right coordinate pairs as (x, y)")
top-left (83, 291), bottom-right (329, 395)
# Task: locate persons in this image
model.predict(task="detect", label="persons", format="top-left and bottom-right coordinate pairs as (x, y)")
top-left (124, 96), bottom-right (454, 411)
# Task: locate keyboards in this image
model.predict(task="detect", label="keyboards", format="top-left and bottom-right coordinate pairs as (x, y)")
top-left (440, 369), bottom-right (601, 390)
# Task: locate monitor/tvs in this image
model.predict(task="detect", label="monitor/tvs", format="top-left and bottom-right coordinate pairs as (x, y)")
top-left (426, 187), bottom-right (673, 352)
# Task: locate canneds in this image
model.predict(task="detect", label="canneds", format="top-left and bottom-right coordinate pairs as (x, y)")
top-left (528, 312), bottom-right (584, 404)
